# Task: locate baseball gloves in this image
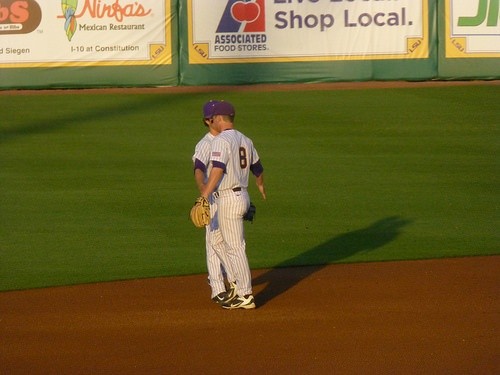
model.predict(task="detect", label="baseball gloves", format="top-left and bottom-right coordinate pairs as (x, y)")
top-left (241, 201), bottom-right (256, 221)
top-left (189, 197), bottom-right (211, 228)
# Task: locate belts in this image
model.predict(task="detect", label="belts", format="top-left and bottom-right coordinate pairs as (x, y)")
top-left (213, 187), bottom-right (241, 198)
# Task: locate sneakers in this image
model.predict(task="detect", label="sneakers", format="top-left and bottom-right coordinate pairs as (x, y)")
top-left (213, 292), bottom-right (227, 303)
top-left (222, 282), bottom-right (239, 304)
top-left (222, 294), bottom-right (256, 309)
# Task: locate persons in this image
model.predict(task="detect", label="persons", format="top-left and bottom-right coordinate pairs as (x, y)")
top-left (190, 102), bottom-right (266, 310)
top-left (193, 100), bottom-right (234, 304)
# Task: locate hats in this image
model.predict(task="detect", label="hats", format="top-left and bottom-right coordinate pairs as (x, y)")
top-left (204, 100), bottom-right (219, 116)
top-left (205, 99), bottom-right (234, 119)
